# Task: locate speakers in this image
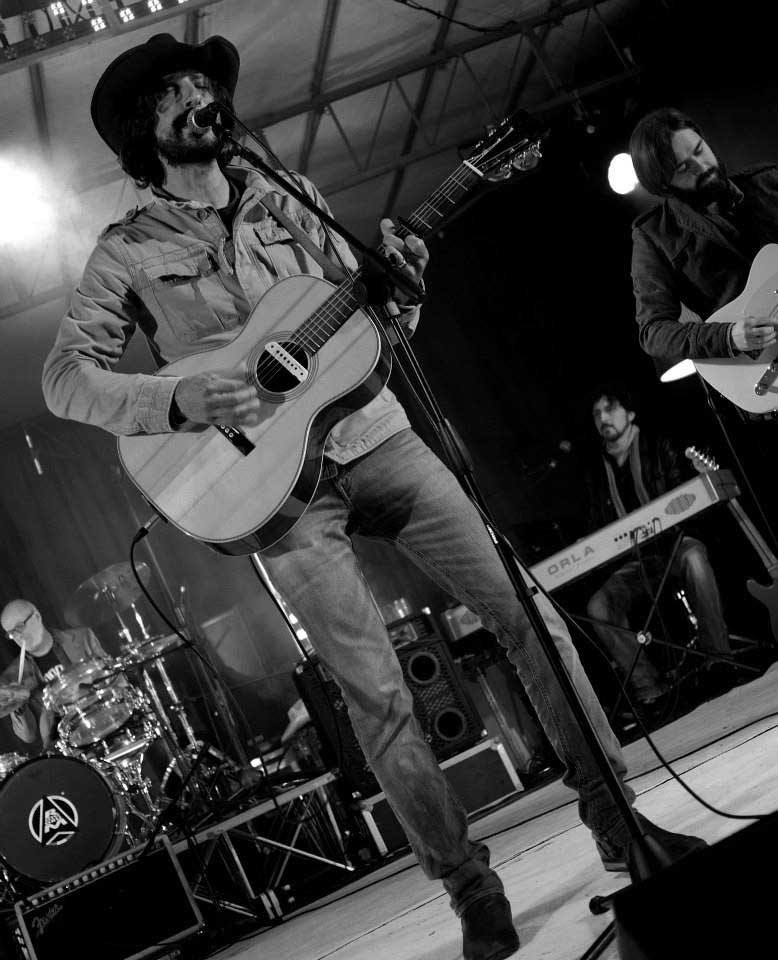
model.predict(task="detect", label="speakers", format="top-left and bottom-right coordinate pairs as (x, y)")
top-left (607, 809), bottom-right (778, 960)
top-left (286, 606), bottom-right (484, 792)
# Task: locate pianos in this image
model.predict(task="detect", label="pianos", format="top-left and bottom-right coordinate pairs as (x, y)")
top-left (439, 472), bottom-right (740, 642)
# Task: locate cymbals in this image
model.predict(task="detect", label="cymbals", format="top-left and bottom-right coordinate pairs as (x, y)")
top-left (1, 686), bottom-right (30, 718)
top-left (128, 631), bottom-right (184, 661)
top-left (63, 560), bottom-right (151, 628)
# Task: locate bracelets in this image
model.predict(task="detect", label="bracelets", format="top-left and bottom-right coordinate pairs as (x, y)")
top-left (15, 703), bottom-right (26, 716)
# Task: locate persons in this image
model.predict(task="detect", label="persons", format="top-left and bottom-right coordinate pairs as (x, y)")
top-left (577, 384), bottom-right (736, 734)
top-left (629, 107), bottom-right (777, 439)
top-left (0, 599), bottom-right (138, 771)
top-left (42, 31), bottom-right (710, 959)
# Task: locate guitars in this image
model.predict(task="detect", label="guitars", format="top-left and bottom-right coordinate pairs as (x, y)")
top-left (696, 240), bottom-right (778, 414)
top-left (684, 445), bottom-right (777, 638)
top-left (115, 113), bottom-right (543, 557)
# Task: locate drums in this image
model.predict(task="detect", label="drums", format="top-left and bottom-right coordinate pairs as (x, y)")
top-left (1, 751), bottom-right (128, 883)
top-left (42, 654), bottom-right (128, 716)
top-left (56, 684), bottom-right (160, 759)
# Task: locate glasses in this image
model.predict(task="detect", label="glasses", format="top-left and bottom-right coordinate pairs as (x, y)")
top-left (4, 610), bottom-right (34, 640)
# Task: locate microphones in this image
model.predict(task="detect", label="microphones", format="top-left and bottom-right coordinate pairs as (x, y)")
top-left (186, 98), bottom-right (230, 136)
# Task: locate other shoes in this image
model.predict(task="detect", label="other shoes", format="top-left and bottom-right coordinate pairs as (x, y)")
top-left (594, 811), bottom-right (708, 872)
top-left (460, 893), bottom-right (520, 960)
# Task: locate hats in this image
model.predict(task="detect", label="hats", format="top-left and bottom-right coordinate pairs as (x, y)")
top-left (90, 32), bottom-right (240, 157)
